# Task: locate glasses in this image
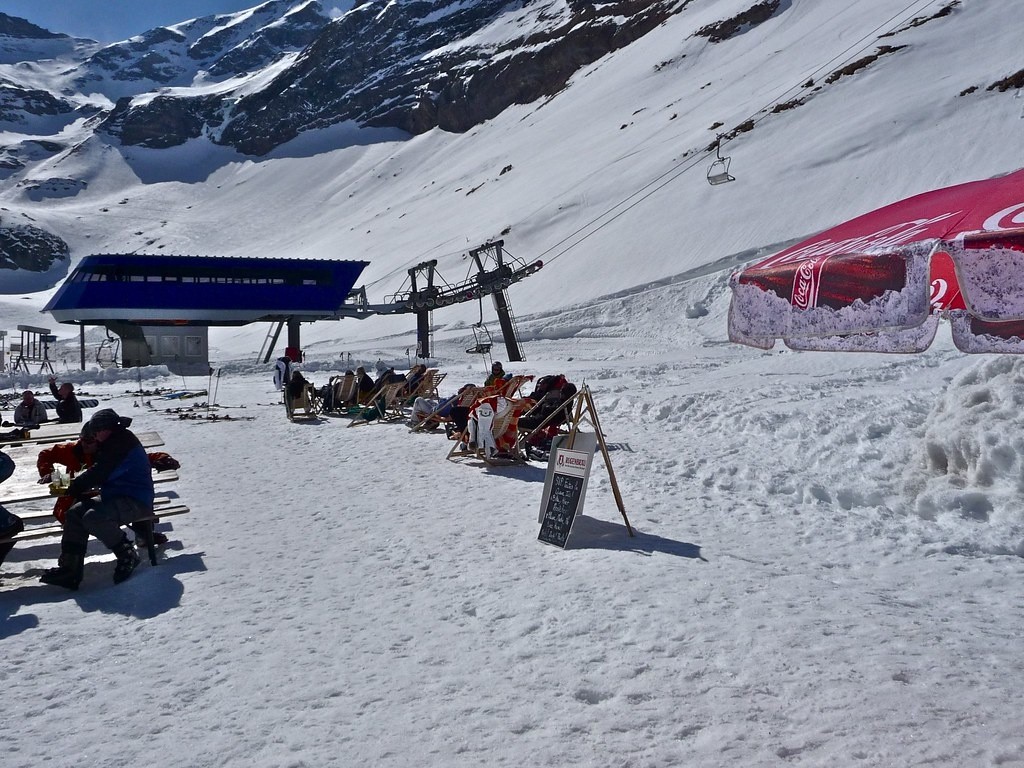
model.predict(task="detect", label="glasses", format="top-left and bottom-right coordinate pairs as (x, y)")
top-left (79, 436), bottom-right (95, 444)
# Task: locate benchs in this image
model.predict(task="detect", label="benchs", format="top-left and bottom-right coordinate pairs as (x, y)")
top-left (0, 423), bottom-right (189, 567)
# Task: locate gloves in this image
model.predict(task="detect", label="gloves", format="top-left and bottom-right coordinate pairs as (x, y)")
top-left (37, 473), bottom-right (53, 484)
top-left (156, 457), bottom-right (181, 474)
top-left (65, 483), bottom-right (79, 498)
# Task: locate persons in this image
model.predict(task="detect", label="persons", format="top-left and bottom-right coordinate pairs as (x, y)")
top-left (14, 390), bottom-right (48, 426)
top-left (48, 377), bottom-right (83, 424)
top-left (37, 420), bottom-right (98, 526)
top-left (292, 359), bottom-right (577, 444)
top-left (39, 408), bottom-right (156, 591)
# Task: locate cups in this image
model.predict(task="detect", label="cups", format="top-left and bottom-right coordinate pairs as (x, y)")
top-left (51, 473), bottom-right (61, 488)
top-left (62, 474), bottom-right (70, 486)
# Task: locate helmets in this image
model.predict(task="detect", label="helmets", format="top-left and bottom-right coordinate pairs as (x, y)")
top-left (491, 362), bottom-right (502, 376)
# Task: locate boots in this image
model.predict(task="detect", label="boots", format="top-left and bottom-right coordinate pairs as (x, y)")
top-left (133, 521), bottom-right (168, 548)
top-left (112, 541), bottom-right (142, 585)
top-left (39, 558), bottom-right (84, 591)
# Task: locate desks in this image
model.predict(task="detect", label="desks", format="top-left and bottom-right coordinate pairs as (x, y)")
top-left (0, 423), bottom-right (84, 445)
top-left (0, 431), bottom-right (164, 463)
top-left (0, 463), bottom-right (178, 504)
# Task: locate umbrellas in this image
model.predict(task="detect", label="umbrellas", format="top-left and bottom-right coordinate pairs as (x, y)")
top-left (724, 167), bottom-right (1024, 359)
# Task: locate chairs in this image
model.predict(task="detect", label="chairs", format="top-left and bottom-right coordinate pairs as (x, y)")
top-left (283, 365), bottom-right (581, 467)
